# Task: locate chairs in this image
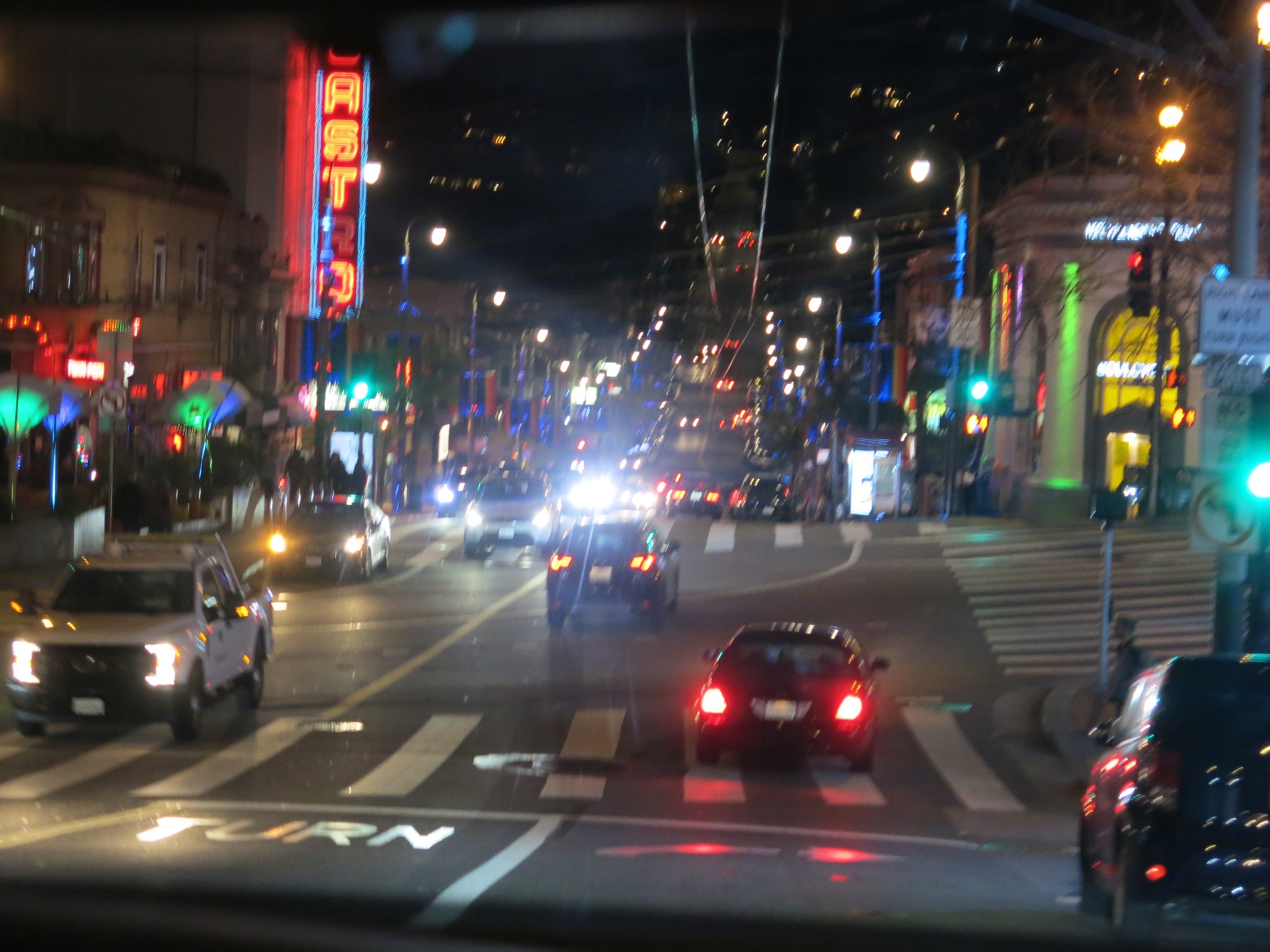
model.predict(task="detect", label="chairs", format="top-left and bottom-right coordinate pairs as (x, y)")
top-left (748, 647), bottom-right (838, 674)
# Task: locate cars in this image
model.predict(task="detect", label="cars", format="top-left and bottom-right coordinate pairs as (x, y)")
top-left (264, 495), bottom-right (395, 583)
top-left (545, 518), bottom-right (683, 631)
top-left (425, 402), bottom-right (809, 571)
top-left (693, 621), bottom-right (892, 775)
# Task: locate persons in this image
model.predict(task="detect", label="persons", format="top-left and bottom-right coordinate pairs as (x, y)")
top-left (1104, 614), bottom-right (1151, 721)
top-left (146, 481), bottom-right (173, 536)
top-left (241, 429), bottom-right (368, 532)
top-left (113, 470), bottom-right (150, 538)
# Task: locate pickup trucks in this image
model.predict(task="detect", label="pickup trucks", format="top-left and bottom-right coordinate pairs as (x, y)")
top-left (2, 530), bottom-right (279, 751)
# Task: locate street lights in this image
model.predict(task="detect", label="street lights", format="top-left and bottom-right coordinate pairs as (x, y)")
top-left (805, 291), bottom-right (847, 510)
top-left (516, 325), bottom-right (550, 433)
top-left (907, 139), bottom-right (979, 524)
top-left (398, 212), bottom-right (450, 513)
top-left (551, 356), bottom-right (572, 445)
top-left (464, 283), bottom-right (508, 466)
top-left (831, 218), bottom-right (882, 440)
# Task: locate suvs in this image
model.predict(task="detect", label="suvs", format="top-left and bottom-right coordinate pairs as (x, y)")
top-left (1078, 641), bottom-right (1270, 925)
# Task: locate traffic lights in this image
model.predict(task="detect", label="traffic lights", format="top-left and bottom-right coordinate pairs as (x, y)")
top-left (1154, 89), bottom-right (1191, 172)
top-left (1230, 400), bottom-right (1270, 537)
top-left (961, 410), bottom-right (989, 436)
top-left (1129, 243), bottom-right (1153, 284)
top-left (964, 371), bottom-right (992, 412)
